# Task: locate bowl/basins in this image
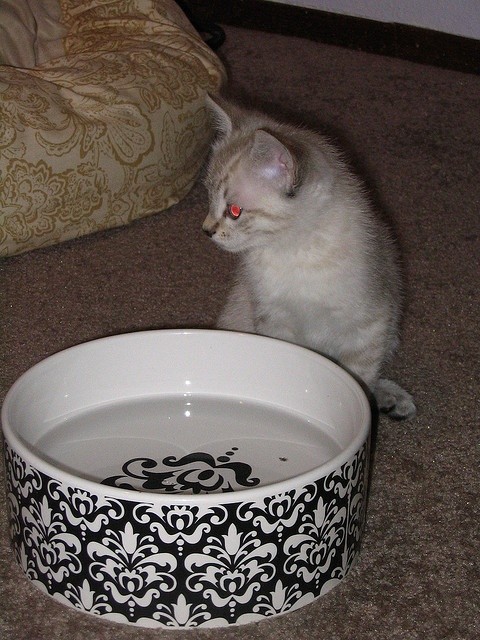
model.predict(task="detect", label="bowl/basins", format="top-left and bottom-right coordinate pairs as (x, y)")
top-left (0, 328), bottom-right (373, 630)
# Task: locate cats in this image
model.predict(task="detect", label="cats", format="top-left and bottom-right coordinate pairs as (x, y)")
top-left (194, 78), bottom-right (419, 423)
